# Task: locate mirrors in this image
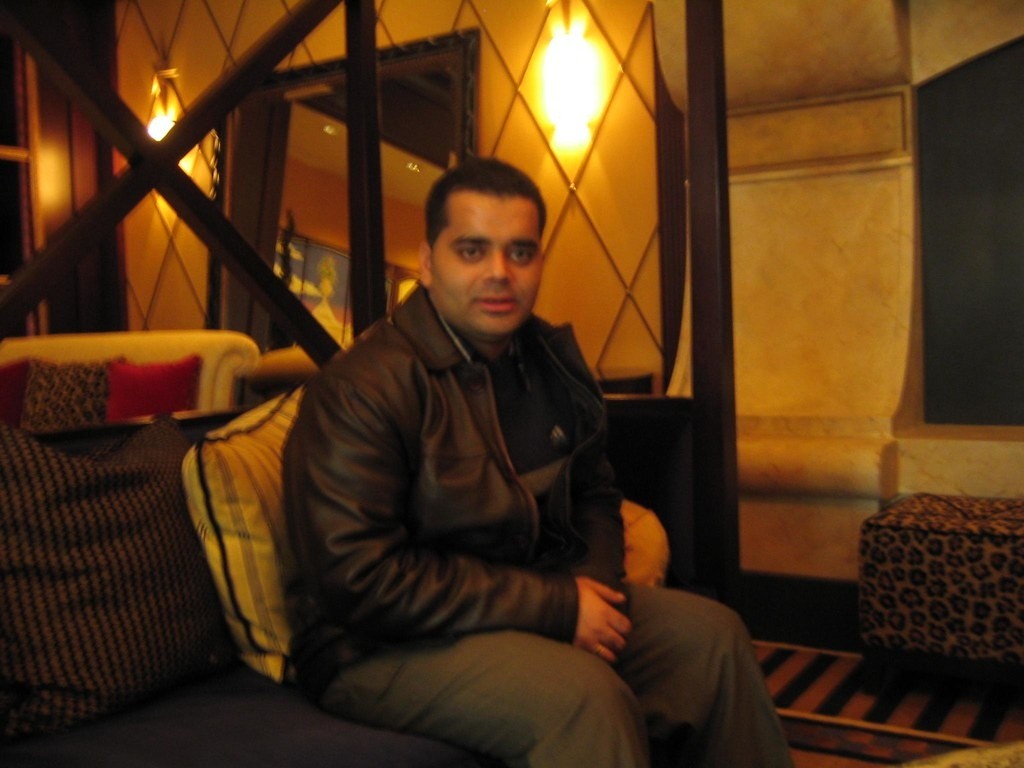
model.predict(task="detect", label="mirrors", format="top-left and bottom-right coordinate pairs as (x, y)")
top-left (210, 22), bottom-right (488, 403)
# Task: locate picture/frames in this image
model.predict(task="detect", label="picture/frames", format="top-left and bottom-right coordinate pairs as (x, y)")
top-left (268, 228), bottom-right (351, 347)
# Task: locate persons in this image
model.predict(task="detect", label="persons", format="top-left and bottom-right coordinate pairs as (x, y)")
top-left (284, 159), bottom-right (791, 767)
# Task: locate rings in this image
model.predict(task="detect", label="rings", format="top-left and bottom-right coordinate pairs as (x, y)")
top-left (596, 644), bottom-right (604, 655)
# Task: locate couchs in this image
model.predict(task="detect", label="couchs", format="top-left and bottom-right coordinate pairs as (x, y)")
top-left (1, 329), bottom-right (256, 423)
top-left (1, 383), bottom-right (689, 767)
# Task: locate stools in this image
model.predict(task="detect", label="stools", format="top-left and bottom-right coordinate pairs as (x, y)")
top-left (862, 490), bottom-right (1024, 666)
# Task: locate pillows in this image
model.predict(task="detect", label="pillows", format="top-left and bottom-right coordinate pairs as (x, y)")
top-left (1, 357), bottom-right (27, 424)
top-left (179, 384), bottom-right (304, 684)
top-left (109, 355), bottom-right (203, 421)
top-left (19, 355), bottom-right (122, 423)
top-left (1, 422), bottom-right (234, 739)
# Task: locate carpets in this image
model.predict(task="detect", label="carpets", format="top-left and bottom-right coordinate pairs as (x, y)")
top-left (736, 565), bottom-right (1023, 768)
